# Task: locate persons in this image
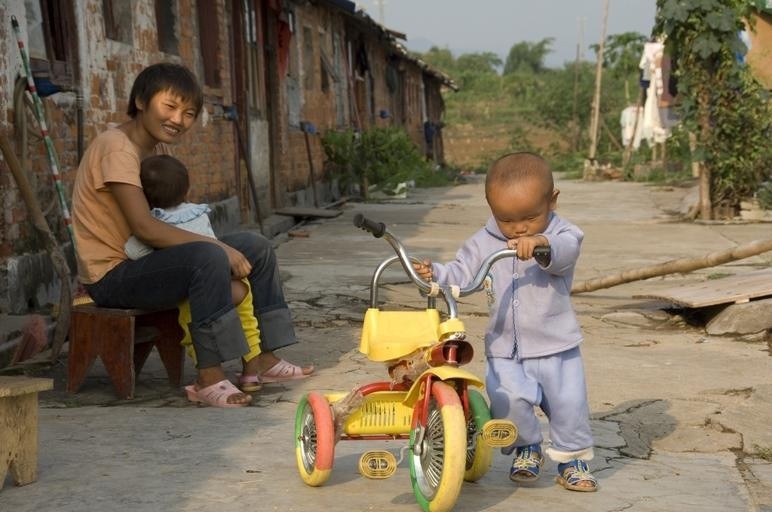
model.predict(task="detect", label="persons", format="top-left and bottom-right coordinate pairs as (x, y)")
top-left (123, 152), bottom-right (265, 396)
top-left (408, 150), bottom-right (605, 491)
top-left (69, 61), bottom-right (318, 410)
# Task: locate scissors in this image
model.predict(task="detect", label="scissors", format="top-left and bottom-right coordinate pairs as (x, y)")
top-left (0, 126), bottom-right (73, 366)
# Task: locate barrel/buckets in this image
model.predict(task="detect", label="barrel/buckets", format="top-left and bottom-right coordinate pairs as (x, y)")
top-left (185, 379), bottom-right (253, 408)
top-left (237, 358), bottom-right (314, 391)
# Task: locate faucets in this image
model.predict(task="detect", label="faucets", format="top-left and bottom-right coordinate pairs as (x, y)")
top-left (510, 444), bottom-right (545, 484)
top-left (555, 458), bottom-right (598, 493)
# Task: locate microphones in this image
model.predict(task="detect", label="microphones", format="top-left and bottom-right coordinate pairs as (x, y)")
top-left (67, 301), bottom-right (187, 400)
top-left (0, 375), bottom-right (53, 490)
